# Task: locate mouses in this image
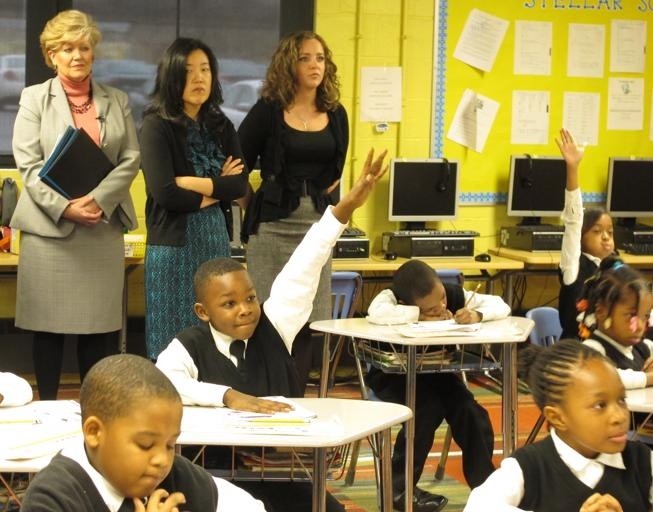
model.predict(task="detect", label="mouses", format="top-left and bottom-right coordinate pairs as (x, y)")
top-left (474, 253), bottom-right (490, 262)
top-left (384, 249), bottom-right (397, 261)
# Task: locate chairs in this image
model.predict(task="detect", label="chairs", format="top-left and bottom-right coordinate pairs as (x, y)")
top-left (329, 271), bottom-right (376, 401)
top-left (520, 306), bottom-right (562, 448)
top-left (343, 264), bottom-right (466, 487)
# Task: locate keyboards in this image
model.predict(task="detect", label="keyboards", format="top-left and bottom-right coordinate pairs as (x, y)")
top-left (340, 227), bottom-right (366, 236)
top-left (620, 240), bottom-right (653, 255)
top-left (392, 230), bottom-right (480, 237)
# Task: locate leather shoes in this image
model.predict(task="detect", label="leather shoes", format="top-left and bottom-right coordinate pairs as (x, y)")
top-left (384, 486), bottom-right (449, 512)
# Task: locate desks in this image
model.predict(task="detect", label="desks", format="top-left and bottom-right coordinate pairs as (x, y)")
top-left (179, 395), bottom-right (412, 512)
top-left (621, 384), bottom-right (653, 432)
top-left (310, 313), bottom-right (537, 510)
top-left (488, 247), bottom-right (653, 462)
top-left (0, 399), bottom-right (85, 512)
top-left (1, 247), bottom-right (146, 353)
top-left (332, 250), bottom-right (524, 397)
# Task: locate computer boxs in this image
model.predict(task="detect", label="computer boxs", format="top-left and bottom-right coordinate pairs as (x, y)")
top-left (613, 230), bottom-right (653, 249)
top-left (332, 240), bottom-right (370, 257)
top-left (499, 226), bottom-right (563, 251)
top-left (388, 237), bottom-right (474, 257)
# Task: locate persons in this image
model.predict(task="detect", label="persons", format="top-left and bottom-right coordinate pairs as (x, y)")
top-left (9, 9), bottom-right (143, 400)
top-left (453, 339), bottom-right (653, 512)
top-left (238, 30), bottom-right (349, 399)
top-left (154, 147), bottom-right (390, 512)
top-left (19, 353), bottom-right (264, 512)
top-left (577, 253), bottom-right (653, 390)
top-left (367, 259), bottom-right (512, 511)
top-left (555, 128), bottom-right (616, 339)
top-left (141, 39), bottom-right (250, 364)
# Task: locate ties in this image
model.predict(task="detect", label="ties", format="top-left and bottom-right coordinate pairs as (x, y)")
top-left (117, 498), bottom-right (145, 512)
top-left (228, 339), bottom-right (246, 375)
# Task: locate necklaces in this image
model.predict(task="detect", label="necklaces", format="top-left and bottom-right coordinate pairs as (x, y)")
top-left (62, 86), bottom-right (92, 114)
top-left (288, 98), bottom-right (322, 131)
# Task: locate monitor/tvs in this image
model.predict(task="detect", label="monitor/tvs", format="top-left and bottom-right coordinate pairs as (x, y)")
top-left (605, 154), bottom-right (653, 229)
top-left (387, 158), bottom-right (460, 230)
top-left (506, 155), bottom-right (567, 227)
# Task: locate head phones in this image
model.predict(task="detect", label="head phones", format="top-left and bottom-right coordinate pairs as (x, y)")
top-left (523, 154), bottom-right (536, 189)
top-left (437, 159), bottom-right (451, 191)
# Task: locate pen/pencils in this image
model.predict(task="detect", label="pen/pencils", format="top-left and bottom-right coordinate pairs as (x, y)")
top-left (464, 284), bottom-right (482, 308)
top-left (0, 419), bottom-right (42, 424)
top-left (248, 419), bottom-right (312, 423)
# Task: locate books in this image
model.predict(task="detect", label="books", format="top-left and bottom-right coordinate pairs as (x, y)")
top-left (398, 319), bottom-right (482, 337)
